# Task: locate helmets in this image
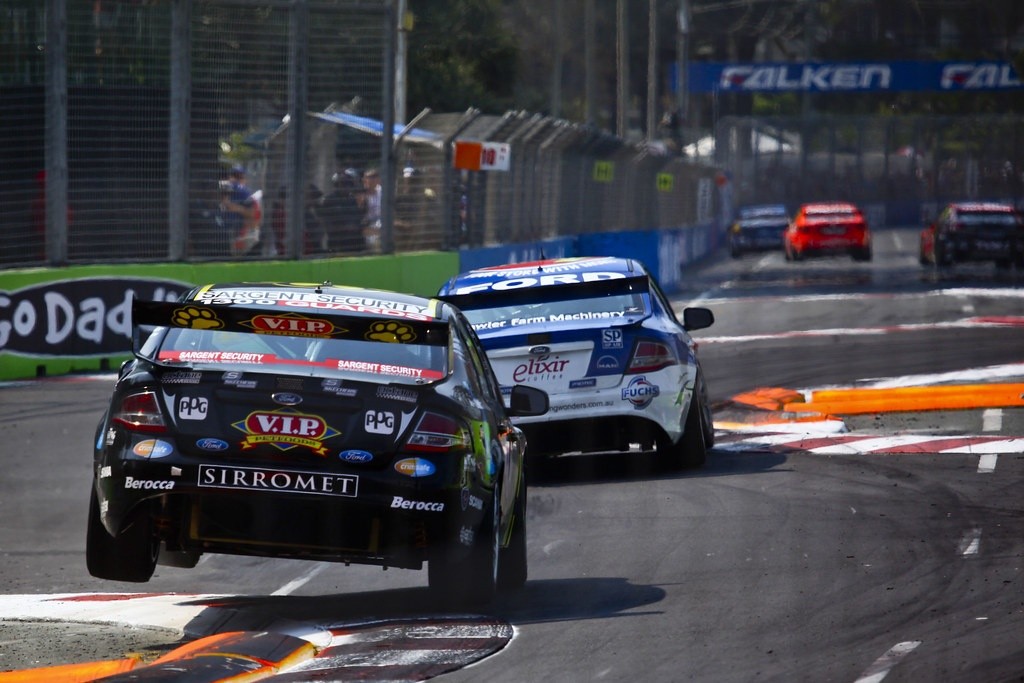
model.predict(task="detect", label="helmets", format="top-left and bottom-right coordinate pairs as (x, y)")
top-left (331, 171), bottom-right (354, 197)
top-left (365, 168), bottom-right (377, 177)
top-left (229, 164), bottom-right (247, 184)
top-left (402, 167), bottom-right (420, 178)
top-left (345, 168), bottom-right (362, 179)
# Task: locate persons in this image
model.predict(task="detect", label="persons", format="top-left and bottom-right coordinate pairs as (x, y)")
top-left (275, 167), bottom-right (432, 254)
top-left (187, 164), bottom-right (259, 256)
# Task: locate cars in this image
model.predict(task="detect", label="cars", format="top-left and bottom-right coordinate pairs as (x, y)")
top-left (728, 201), bottom-right (795, 259)
top-left (783, 200), bottom-right (874, 263)
top-left (428, 254), bottom-right (719, 476)
top-left (918, 199), bottom-right (1024, 273)
top-left (84, 282), bottom-right (555, 619)
